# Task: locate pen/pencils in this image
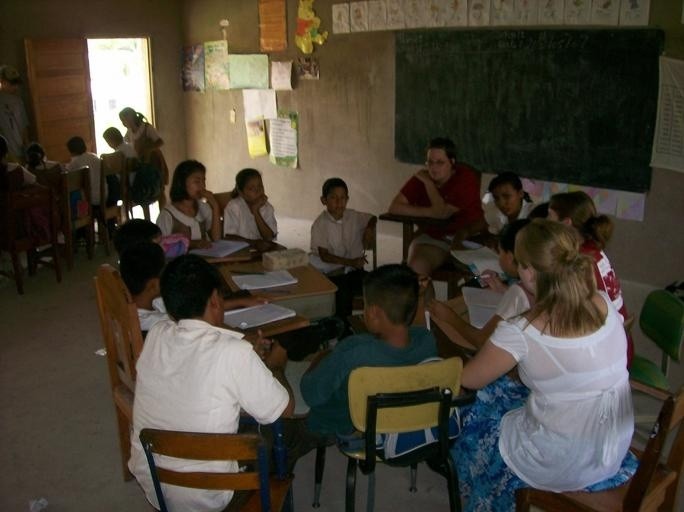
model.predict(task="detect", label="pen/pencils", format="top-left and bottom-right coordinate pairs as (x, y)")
top-left (230, 270), bottom-right (266, 275)
top-left (472, 273), bottom-right (498, 280)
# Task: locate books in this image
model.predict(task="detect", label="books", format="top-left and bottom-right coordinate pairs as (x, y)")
top-left (189, 239), bottom-right (249, 258)
top-left (223, 302), bottom-right (295, 331)
top-left (231, 268), bottom-right (299, 291)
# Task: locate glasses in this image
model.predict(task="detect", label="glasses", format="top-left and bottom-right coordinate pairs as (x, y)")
top-left (424, 158), bottom-right (450, 168)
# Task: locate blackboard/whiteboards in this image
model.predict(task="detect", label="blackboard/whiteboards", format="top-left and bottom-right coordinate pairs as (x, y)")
top-left (395, 27), bottom-right (660, 192)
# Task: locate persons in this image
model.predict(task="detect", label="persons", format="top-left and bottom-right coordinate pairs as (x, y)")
top-left (309, 176), bottom-right (380, 340)
top-left (292, 261), bottom-right (438, 462)
top-left (223, 167), bottom-right (279, 252)
top-left (157, 158), bottom-right (222, 242)
top-left (0, 106), bottom-right (170, 279)
top-left (118, 242), bottom-right (170, 340)
top-left (390, 135), bottom-right (539, 326)
top-left (124, 254), bottom-right (296, 512)
top-left (445, 190), bottom-right (637, 512)
